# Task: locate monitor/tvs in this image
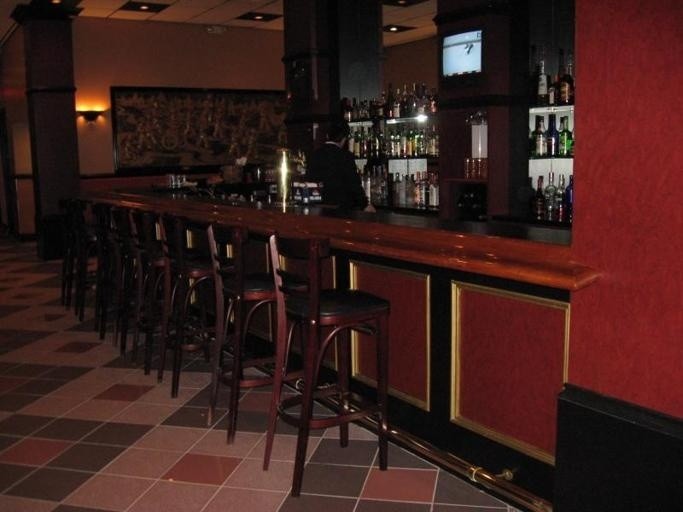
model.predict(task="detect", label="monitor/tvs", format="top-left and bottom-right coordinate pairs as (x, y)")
top-left (437, 26), bottom-right (486, 89)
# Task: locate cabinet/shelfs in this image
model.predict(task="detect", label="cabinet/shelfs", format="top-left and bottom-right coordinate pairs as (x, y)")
top-left (341, 109), bottom-right (439, 215)
top-left (519, 95), bottom-right (574, 229)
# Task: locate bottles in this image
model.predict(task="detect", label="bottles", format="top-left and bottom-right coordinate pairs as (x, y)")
top-left (545, 173), bottom-right (557, 221)
top-left (537, 47), bottom-right (573, 105)
top-left (559, 116), bottom-right (571, 156)
top-left (567, 175), bottom-right (572, 204)
top-left (343, 82), bottom-right (439, 122)
top-left (535, 176), bottom-right (544, 221)
top-left (557, 174), bottom-right (566, 222)
top-left (535, 116), bottom-right (547, 157)
top-left (547, 114), bottom-right (558, 157)
top-left (349, 125), bottom-right (440, 157)
top-left (365, 164), bottom-right (440, 210)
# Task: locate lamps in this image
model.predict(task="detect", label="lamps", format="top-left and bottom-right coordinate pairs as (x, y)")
top-left (75, 110), bottom-right (106, 128)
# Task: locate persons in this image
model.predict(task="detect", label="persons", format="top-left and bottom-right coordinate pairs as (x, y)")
top-left (305, 116), bottom-right (377, 214)
top-left (116, 91), bottom-right (290, 166)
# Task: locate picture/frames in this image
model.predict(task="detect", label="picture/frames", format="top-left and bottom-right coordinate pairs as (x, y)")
top-left (109, 86), bottom-right (289, 180)
